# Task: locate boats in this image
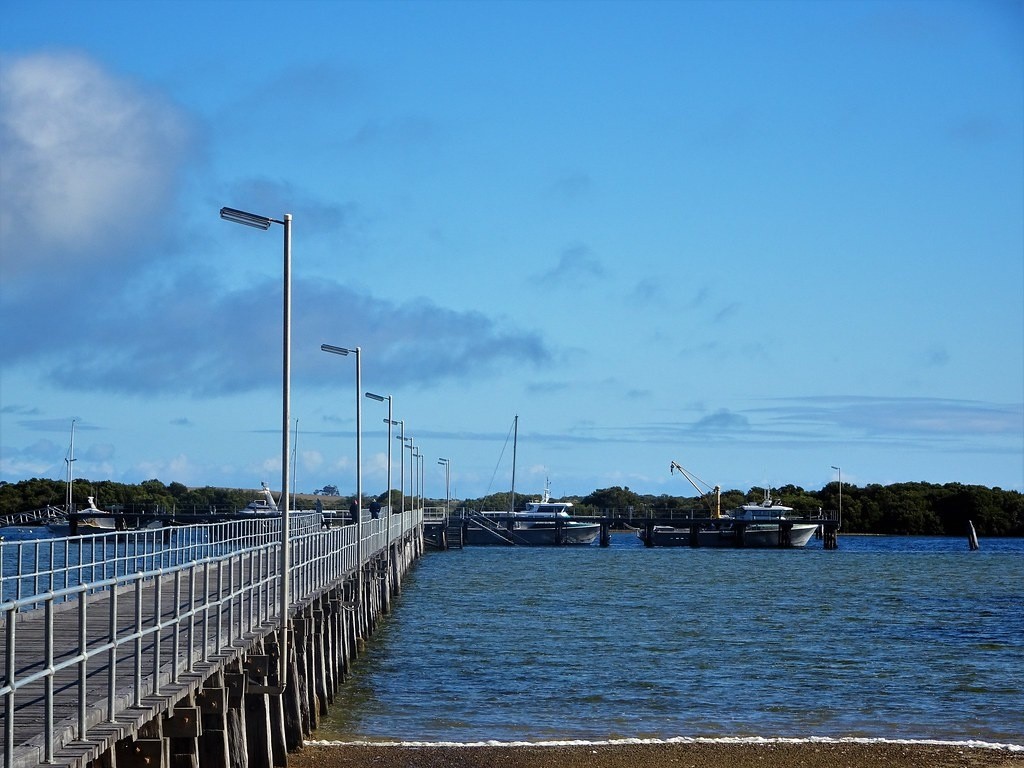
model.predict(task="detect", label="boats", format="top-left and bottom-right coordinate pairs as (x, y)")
top-left (636, 484), bottom-right (820, 547)
top-left (239, 478), bottom-right (337, 519)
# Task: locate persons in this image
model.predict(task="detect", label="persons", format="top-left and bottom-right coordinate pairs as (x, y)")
top-left (350, 500), bottom-right (359, 524)
top-left (369, 499), bottom-right (381, 518)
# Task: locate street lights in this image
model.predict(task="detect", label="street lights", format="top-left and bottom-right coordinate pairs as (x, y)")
top-left (831, 465), bottom-right (842, 528)
top-left (413, 453), bottom-right (425, 532)
top-left (404, 444), bottom-right (419, 538)
top-left (437, 457), bottom-right (450, 527)
top-left (319, 342), bottom-right (363, 607)
top-left (396, 435), bottom-right (415, 544)
top-left (383, 418), bottom-right (406, 555)
top-left (365, 390), bottom-right (394, 574)
top-left (221, 205), bottom-right (294, 696)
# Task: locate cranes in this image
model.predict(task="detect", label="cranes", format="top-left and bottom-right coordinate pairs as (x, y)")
top-left (670, 460), bottom-right (733, 530)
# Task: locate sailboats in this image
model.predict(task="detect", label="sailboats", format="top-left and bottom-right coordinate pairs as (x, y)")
top-left (38, 419), bottom-right (179, 542)
top-left (967, 519), bottom-right (979, 551)
top-left (465, 414), bottom-right (601, 545)
